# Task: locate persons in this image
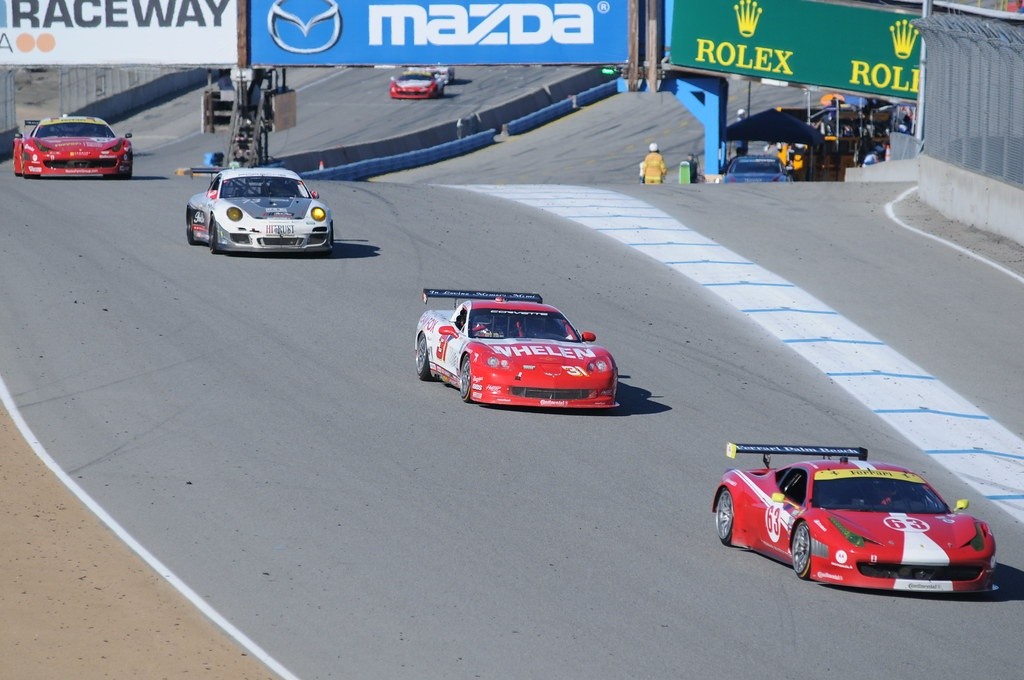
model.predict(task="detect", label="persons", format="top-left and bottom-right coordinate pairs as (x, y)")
top-left (857, 128), bottom-right (874, 167)
top-left (682, 153), bottom-right (697, 183)
top-left (725, 108), bottom-right (748, 174)
top-left (639, 143), bottom-right (668, 185)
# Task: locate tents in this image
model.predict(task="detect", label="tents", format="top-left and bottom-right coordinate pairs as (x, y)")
top-left (727, 108), bottom-right (826, 181)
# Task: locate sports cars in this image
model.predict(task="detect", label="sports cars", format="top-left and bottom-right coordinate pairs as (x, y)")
top-left (413, 287), bottom-right (621, 410)
top-left (13, 113), bottom-right (134, 181)
top-left (174, 163), bottom-right (334, 255)
top-left (709, 442), bottom-right (1001, 596)
top-left (405, 64), bottom-right (455, 86)
top-left (389, 68), bottom-right (445, 99)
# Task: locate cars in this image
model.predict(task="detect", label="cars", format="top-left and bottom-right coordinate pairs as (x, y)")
top-left (718, 151), bottom-right (796, 184)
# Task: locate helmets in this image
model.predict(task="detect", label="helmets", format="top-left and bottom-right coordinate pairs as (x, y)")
top-left (270, 179), bottom-right (287, 194)
top-left (736, 109), bottom-right (746, 116)
top-left (649, 143), bottom-right (658, 152)
top-left (473, 314), bottom-right (492, 326)
top-left (873, 479), bottom-right (896, 504)
